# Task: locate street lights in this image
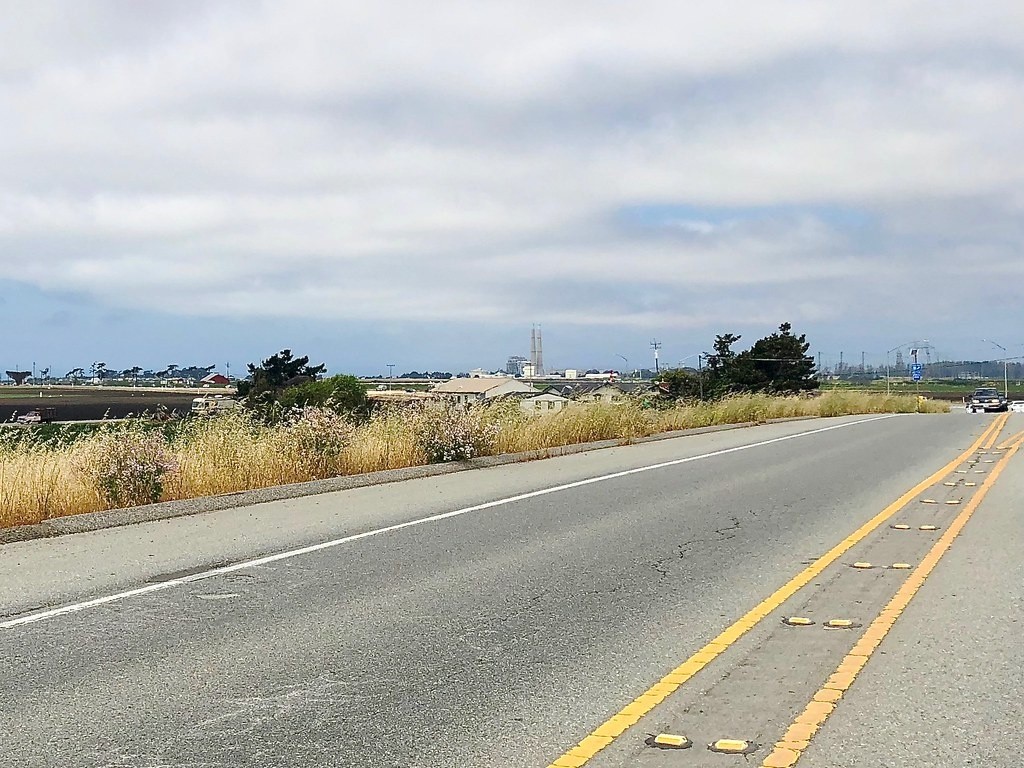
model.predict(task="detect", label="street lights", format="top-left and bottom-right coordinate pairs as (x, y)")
top-left (982, 338), bottom-right (1007, 399)
top-left (886, 340), bottom-right (920, 394)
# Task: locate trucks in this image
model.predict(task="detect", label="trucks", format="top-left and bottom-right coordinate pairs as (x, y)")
top-left (18, 407), bottom-right (56, 424)
top-left (192, 397), bottom-right (235, 415)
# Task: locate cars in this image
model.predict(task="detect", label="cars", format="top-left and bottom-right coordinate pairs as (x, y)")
top-left (969, 388), bottom-right (1011, 412)
